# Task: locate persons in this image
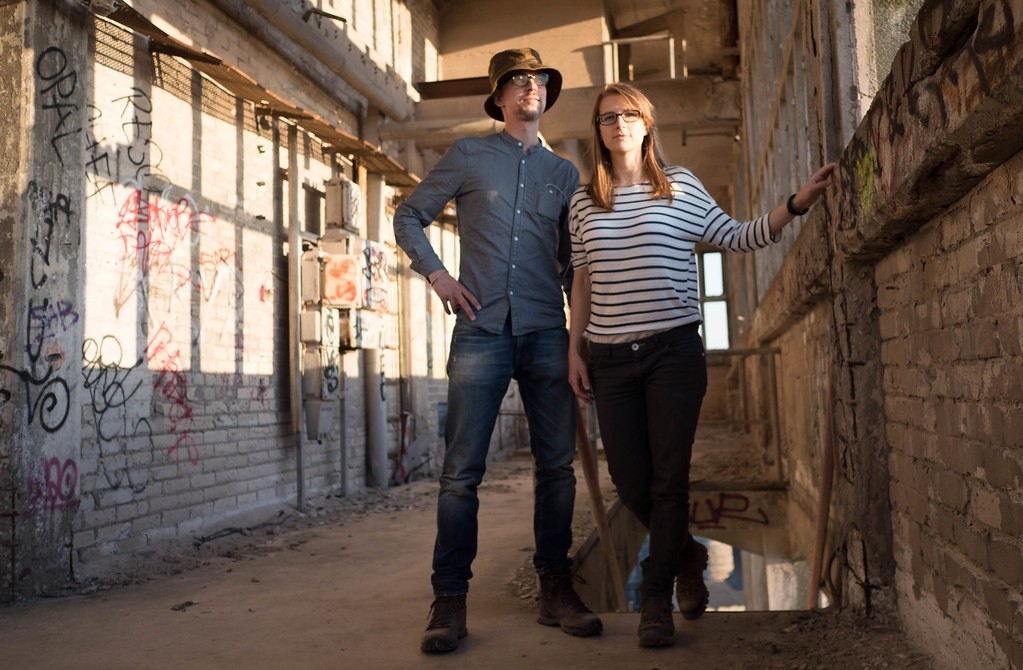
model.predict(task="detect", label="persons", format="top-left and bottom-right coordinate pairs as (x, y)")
top-left (392, 46), bottom-right (602, 650)
top-left (564, 83), bottom-right (837, 649)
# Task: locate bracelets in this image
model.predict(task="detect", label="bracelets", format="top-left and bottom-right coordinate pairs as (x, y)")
top-left (786, 193), bottom-right (810, 215)
top-left (431, 270), bottom-right (448, 288)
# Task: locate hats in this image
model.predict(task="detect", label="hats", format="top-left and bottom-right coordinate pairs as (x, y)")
top-left (483, 47), bottom-right (563, 122)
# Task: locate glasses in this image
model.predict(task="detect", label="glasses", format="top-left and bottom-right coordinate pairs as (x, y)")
top-left (596, 110), bottom-right (642, 126)
top-left (501, 72), bottom-right (549, 86)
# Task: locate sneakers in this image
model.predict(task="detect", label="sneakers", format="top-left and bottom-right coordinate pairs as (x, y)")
top-left (637, 600), bottom-right (676, 650)
top-left (420, 593), bottom-right (468, 656)
top-left (673, 539), bottom-right (709, 619)
top-left (537, 568), bottom-right (603, 637)
